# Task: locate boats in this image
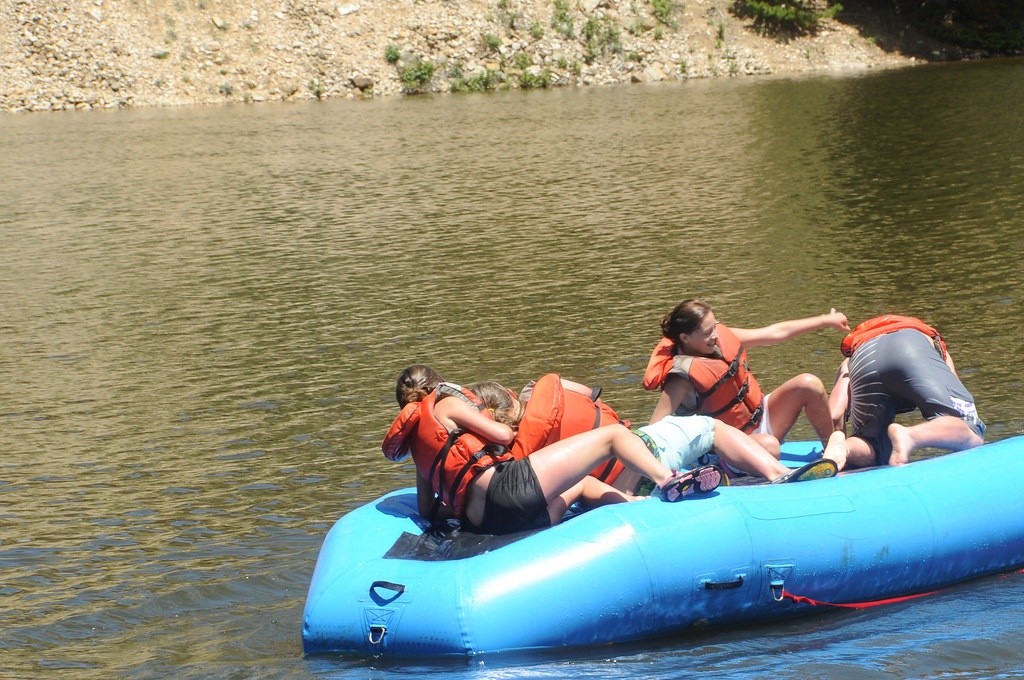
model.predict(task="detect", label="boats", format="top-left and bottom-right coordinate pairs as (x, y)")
top-left (297, 437), bottom-right (1021, 665)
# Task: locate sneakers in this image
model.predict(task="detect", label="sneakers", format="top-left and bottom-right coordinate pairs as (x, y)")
top-left (773, 458), bottom-right (839, 486)
top-left (659, 465), bottom-right (723, 503)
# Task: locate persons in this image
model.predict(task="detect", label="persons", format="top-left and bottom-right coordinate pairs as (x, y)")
top-left (381, 366), bottom-right (723, 536)
top-left (641, 298), bottom-right (851, 475)
top-left (469, 372), bottom-right (838, 499)
top-left (821, 314), bottom-right (987, 471)
